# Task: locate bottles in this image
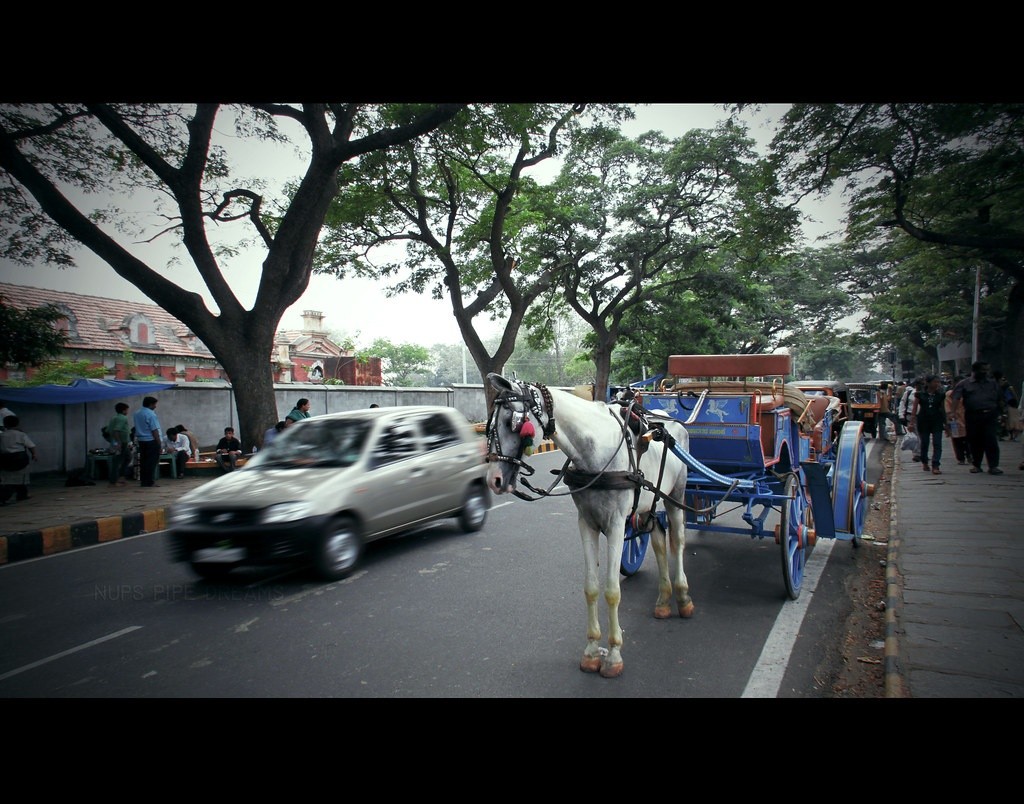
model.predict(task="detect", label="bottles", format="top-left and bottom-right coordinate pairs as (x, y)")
top-left (252, 445), bottom-right (258, 453)
top-left (950, 417), bottom-right (959, 438)
top-left (194, 448), bottom-right (200, 462)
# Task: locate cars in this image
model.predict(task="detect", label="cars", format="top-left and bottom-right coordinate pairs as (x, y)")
top-left (164, 405), bottom-right (494, 588)
top-left (782, 372), bottom-right (889, 445)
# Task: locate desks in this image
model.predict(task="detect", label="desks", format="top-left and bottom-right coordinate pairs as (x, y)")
top-left (154, 454), bottom-right (177, 479)
top-left (86, 454), bottom-right (117, 483)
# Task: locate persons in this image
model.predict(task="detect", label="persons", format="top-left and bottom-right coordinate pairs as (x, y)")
top-left (107, 402), bottom-right (133, 483)
top-left (175, 425), bottom-right (199, 457)
top-left (214, 427), bottom-right (242, 472)
top-left (161, 427), bottom-right (192, 478)
top-left (134, 396), bottom-right (162, 486)
top-left (877, 360), bottom-right (1024, 476)
top-left (0, 415), bottom-right (37, 507)
top-left (262, 421), bottom-right (287, 449)
top-left (286, 397), bottom-right (312, 426)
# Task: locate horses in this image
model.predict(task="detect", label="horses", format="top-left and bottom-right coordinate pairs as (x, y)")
top-left (485, 373), bottom-right (695, 679)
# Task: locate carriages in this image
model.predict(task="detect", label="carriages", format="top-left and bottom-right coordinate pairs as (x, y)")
top-left (480, 344), bottom-right (882, 680)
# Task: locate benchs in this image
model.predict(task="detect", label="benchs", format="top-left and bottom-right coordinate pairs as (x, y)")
top-left (200, 451), bottom-right (217, 458)
top-left (184, 459), bottom-right (247, 468)
top-left (754, 394), bottom-right (784, 411)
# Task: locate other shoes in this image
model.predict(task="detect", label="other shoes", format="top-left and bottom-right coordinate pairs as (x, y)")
top-left (178, 474), bottom-right (184, 479)
top-left (922, 465), bottom-right (931, 470)
top-left (106, 480), bottom-right (127, 487)
top-left (969, 467), bottom-right (983, 473)
top-left (18, 493), bottom-right (33, 499)
top-left (1, 499), bottom-right (9, 505)
top-left (142, 482), bottom-right (160, 486)
top-left (988, 468), bottom-right (1003, 474)
top-left (932, 467), bottom-right (941, 474)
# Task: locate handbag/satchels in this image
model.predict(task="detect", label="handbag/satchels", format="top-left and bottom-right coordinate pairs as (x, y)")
top-left (899, 431), bottom-right (917, 451)
top-left (1, 451), bottom-right (30, 472)
top-left (897, 411), bottom-right (908, 426)
